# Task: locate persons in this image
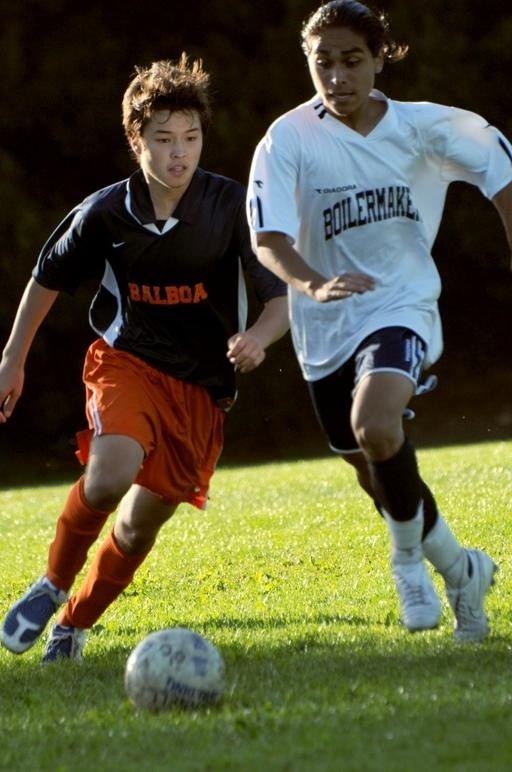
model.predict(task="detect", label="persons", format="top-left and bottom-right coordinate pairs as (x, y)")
top-left (246, 0), bottom-right (511, 642)
top-left (0, 52), bottom-right (293, 668)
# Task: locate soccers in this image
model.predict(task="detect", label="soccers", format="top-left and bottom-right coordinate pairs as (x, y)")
top-left (121, 628), bottom-right (229, 716)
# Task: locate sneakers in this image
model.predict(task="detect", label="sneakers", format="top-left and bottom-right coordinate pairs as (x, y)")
top-left (391, 557), bottom-right (441, 632)
top-left (446, 549), bottom-right (498, 643)
top-left (1, 573), bottom-right (85, 663)
